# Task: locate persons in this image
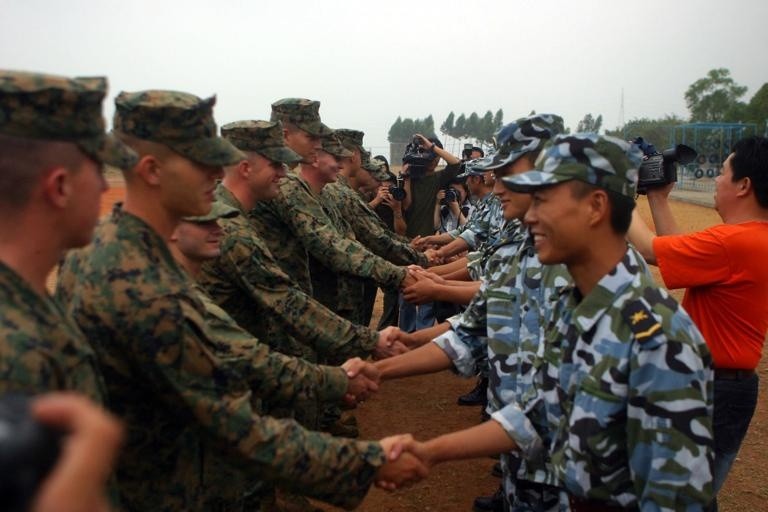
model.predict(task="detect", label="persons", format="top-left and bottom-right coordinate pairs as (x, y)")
top-left (29, 388), bottom-right (122, 511)
top-left (53, 89), bottom-right (431, 512)
top-left (626, 137), bottom-right (768, 493)
top-left (0, 67), bottom-right (123, 512)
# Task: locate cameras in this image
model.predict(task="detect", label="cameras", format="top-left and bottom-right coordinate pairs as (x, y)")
top-left (383, 187), bottom-right (407, 202)
top-left (440, 189), bottom-right (460, 205)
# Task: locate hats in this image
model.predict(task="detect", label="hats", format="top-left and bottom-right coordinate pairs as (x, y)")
top-left (362, 150), bottom-right (390, 182)
top-left (471, 113), bottom-right (566, 168)
top-left (473, 151), bottom-right (495, 174)
top-left (455, 158), bottom-right (481, 177)
top-left (0, 68), bottom-right (141, 169)
top-left (221, 119), bottom-right (303, 164)
top-left (332, 128), bottom-right (368, 154)
top-left (320, 123), bottom-right (352, 157)
top-left (502, 132), bottom-right (645, 200)
top-left (109, 89), bottom-right (247, 171)
top-left (269, 99), bottom-right (334, 140)
